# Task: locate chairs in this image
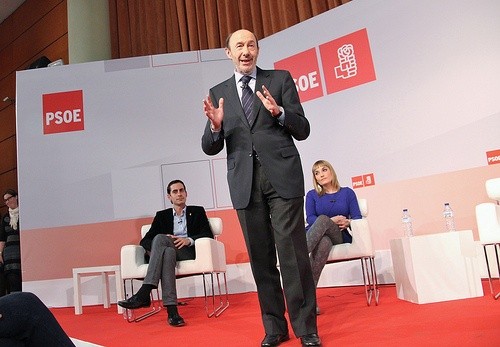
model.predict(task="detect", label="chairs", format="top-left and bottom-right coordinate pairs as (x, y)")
top-left (304, 196), bottom-right (380, 307)
top-left (475, 177), bottom-right (500, 300)
top-left (121, 217), bottom-right (230, 323)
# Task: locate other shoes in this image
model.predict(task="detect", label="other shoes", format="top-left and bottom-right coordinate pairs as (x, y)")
top-left (117, 294), bottom-right (151, 309)
top-left (166, 306), bottom-right (185, 327)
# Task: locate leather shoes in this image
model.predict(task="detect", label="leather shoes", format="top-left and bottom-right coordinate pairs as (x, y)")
top-left (300, 332), bottom-right (321, 347)
top-left (261, 334), bottom-right (289, 346)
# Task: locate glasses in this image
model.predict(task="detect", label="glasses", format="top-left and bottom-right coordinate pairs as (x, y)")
top-left (241, 76), bottom-right (256, 151)
top-left (3, 195), bottom-right (13, 203)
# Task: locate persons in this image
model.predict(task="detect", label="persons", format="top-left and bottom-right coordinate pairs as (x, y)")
top-left (305, 160), bottom-right (362, 315)
top-left (202, 29), bottom-right (321, 347)
top-left (0, 188), bottom-right (22, 291)
top-left (0, 292), bottom-right (77, 347)
top-left (118, 180), bottom-right (214, 326)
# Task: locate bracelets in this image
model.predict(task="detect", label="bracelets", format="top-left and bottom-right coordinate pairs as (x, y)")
top-left (0, 252), bottom-right (2, 254)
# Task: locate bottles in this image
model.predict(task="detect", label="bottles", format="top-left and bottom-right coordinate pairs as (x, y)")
top-left (442, 203), bottom-right (455, 231)
top-left (401, 209), bottom-right (413, 237)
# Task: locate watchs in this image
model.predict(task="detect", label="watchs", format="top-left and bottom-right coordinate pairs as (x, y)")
top-left (272, 106), bottom-right (284, 120)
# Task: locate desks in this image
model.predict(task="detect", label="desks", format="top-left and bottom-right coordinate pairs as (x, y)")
top-left (72, 265), bottom-right (124, 314)
top-left (391, 229), bottom-right (485, 304)
